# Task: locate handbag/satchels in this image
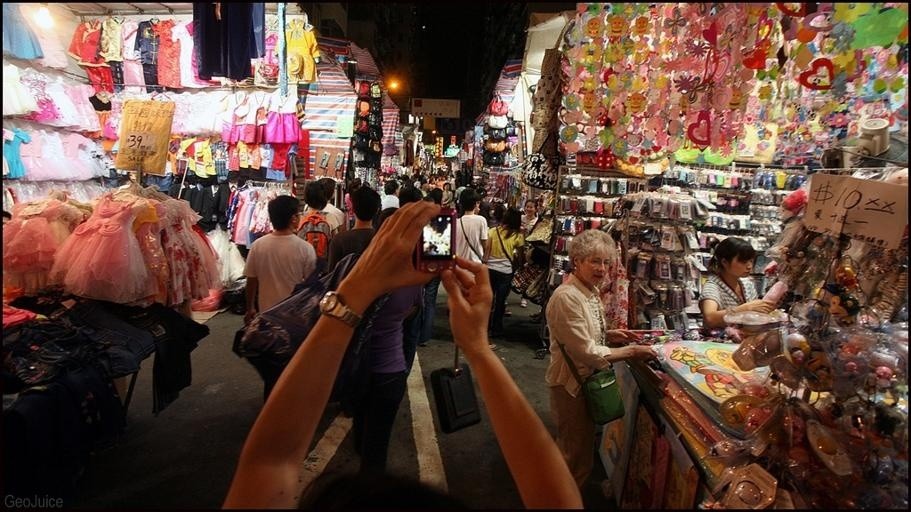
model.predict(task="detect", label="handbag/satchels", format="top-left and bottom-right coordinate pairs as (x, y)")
top-left (481, 95), bottom-right (519, 167)
top-left (511, 215), bottom-right (554, 307)
top-left (521, 48), bottom-right (567, 189)
top-left (580, 369), bottom-right (625, 425)
top-left (352, 81), bottom-right (383, 163)
top-left (239, 252), bottom-right (362, 384)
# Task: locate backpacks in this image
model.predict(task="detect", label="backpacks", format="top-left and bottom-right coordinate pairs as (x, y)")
top-left (296, 211), bottom-right (337, 270)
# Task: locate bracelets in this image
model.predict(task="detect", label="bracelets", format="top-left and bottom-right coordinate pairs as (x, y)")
top-left (724, 307), bottom-right (736, 318)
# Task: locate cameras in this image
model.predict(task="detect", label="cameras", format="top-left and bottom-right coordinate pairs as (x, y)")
top-left (417, 207), bottom-right (456, 277)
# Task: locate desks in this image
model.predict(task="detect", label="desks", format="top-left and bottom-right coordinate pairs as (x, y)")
top-left (596, 342), bottom-right (736, 497)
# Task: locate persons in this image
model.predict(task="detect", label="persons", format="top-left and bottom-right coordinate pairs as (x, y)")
top-left (218, 199), bottom-right (586, 508)
top-left (245, 169), bottom-right (547, 473)
top-left (540, 227), bottom-right (660, 491)
top-left (697, 235), bottom-right (778, 328)
